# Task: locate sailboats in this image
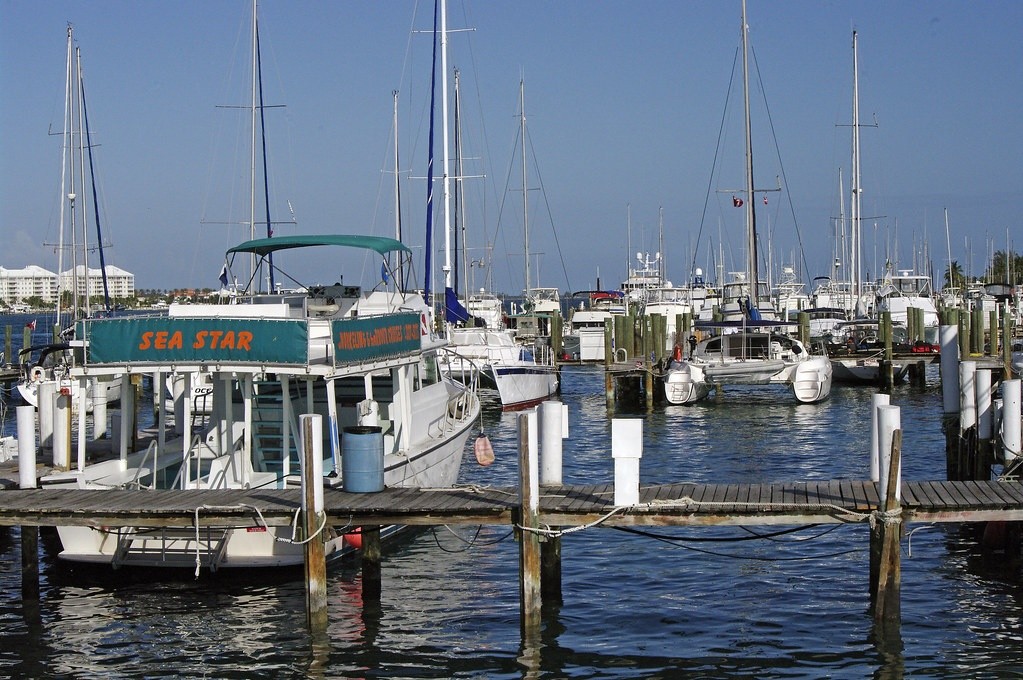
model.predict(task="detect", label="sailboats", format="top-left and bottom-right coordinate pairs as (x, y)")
top-left (1, 1), bottom-right (1022, 578)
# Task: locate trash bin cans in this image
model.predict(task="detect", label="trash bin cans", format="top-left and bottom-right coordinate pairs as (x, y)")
top-left (341, 425), bottom-right (384, 494)
